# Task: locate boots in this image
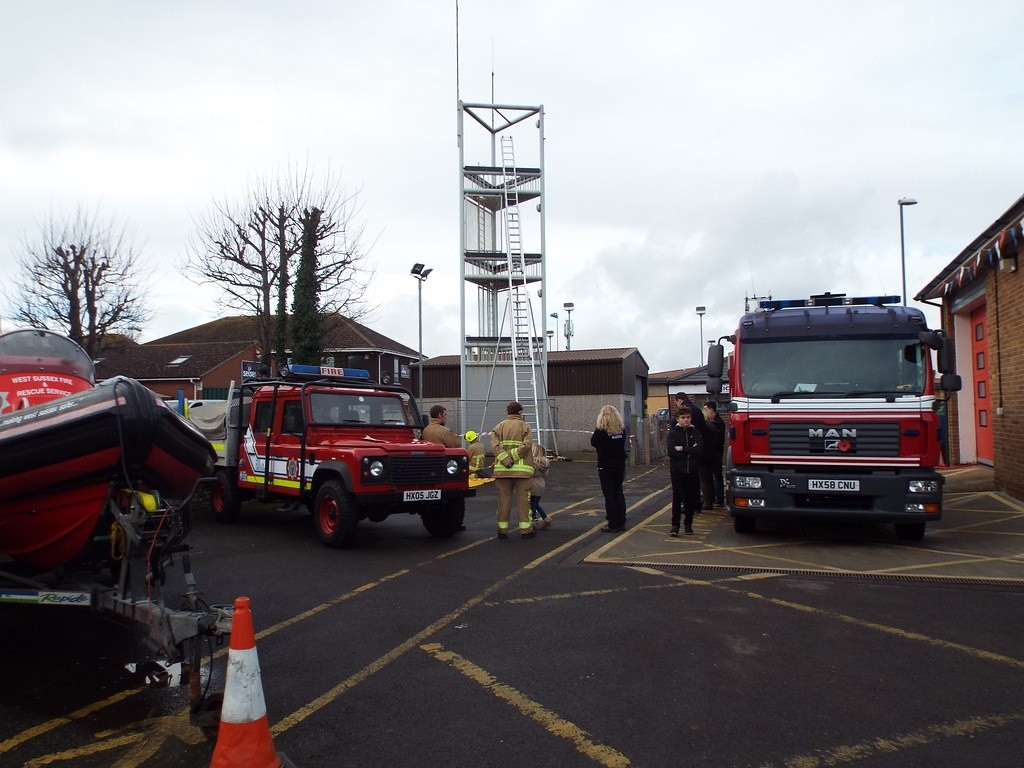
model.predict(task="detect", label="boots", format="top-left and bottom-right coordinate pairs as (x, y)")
top-left (533, 517), bottom-right (551, 528)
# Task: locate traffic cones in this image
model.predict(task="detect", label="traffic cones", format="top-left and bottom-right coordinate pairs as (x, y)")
top-left (210, 597), bottom-right (287, 768)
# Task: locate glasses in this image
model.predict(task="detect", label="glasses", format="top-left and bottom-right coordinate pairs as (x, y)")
top-left (441, 412), bottom-right (448, 416)
top-left (679, 415), bottom-right (691, 419)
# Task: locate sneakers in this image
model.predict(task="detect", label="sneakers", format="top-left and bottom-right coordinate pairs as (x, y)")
top-left (684, 525), bottom-right (693, 535)
top-left (669, 525), bottom-right (679, 536)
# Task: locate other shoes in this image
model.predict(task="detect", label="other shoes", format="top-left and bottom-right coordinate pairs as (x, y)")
top-left (680, 506), bottom-right (702, 514)
top-left (702, 501), bottom-right (714, 511)
top-left (944, 462), bottom-right (950, 466)
top-left (715, 498), bottom-right (725, 507)
top-left (522, 531), bottom-right (536, 538)
top-left (498, 533), bottom-right (507, 539)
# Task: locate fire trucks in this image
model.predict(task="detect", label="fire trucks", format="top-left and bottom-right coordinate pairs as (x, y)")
top-left (708, 292), bottom-right (962, 538)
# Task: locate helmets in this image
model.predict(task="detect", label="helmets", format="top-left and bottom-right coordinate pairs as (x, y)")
top-left (465, 431), bottom-right (478, 441)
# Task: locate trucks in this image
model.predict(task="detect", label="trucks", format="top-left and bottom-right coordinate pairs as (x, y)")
top-left (179, 363), bottom-right (478, 545)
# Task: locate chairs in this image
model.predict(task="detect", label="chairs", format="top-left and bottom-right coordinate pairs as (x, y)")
top-left (285, 408), bottom-right (303, 434)
top-left (338, 410), bottom-right (359, 424)
top-left (784, 344), bottom-right (823, 393)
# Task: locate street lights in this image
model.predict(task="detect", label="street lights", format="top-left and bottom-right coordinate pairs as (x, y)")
top-left (897, 197), bottom-right (917, 306)
top-left (547, 330), bottom-right (554, 352)
top-left (550, 313), bottom-right (559, 350)
top-left (411, 263), bottom-right (434, 420)
top-left (563, 303), bottom-right (575, 349)
top-left (696, 307), bottom-right (706, 367)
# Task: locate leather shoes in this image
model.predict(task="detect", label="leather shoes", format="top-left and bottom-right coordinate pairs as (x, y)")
top-left (601, 523), bottom-right (626, 533)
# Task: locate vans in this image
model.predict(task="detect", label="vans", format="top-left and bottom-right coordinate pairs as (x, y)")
top-left (164, 400), bottom-right (226, 411)
top-left (656, 408), bottom-right (669, 422)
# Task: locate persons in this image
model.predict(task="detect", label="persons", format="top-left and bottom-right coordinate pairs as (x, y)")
top-left (668, 392), bottom-right (725, 512)
top-left (422, 405), bottom-right (467, 530)
top-left (528, 444), bottom-right (552, 530)
top-left (463, 431), bottom-right (485, 474)
top-left (490, 402), bottom-right (537, 538)
top-left (666, 407), bottom-right (704, 536)
top-left (591, 405), bottom-right (628, 532)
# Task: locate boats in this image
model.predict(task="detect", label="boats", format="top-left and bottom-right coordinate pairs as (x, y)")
top-left (0, 328), bottom-right (219, 583)
